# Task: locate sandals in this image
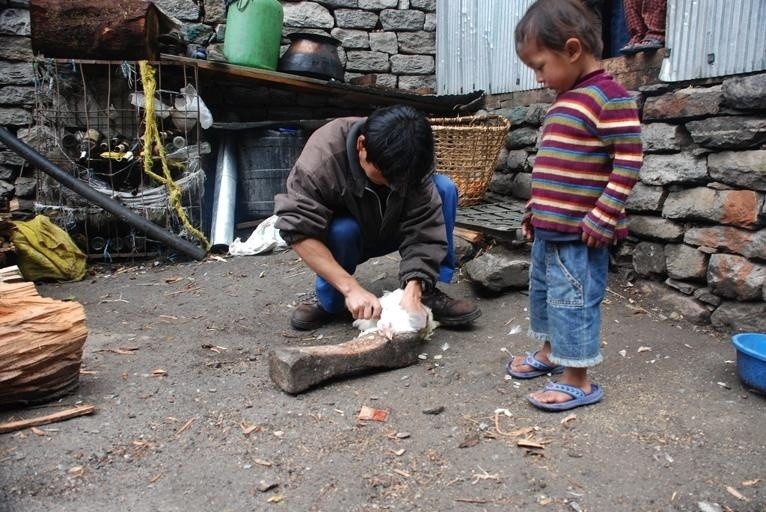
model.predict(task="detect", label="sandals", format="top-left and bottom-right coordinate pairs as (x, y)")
top-left (619, 44), bottom-right (635, 52)
top-left (634, 38), bottom-right (663, 50)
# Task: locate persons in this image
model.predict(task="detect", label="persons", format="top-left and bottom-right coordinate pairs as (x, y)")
top-left (619, 0), bottom-right (667, 52)
top-left (504, 0), bottom-right (645, 411)
top-left (271, 103), bottom-right (483, 331)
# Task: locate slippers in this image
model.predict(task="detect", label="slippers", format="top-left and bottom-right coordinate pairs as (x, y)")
top-left (527, 382), bottom-right (603, 411)
top-left (506, 351), bottom-right (563, 378)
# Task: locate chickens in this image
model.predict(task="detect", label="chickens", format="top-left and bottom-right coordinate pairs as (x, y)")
top-left (352, 288), bottom-right (440, 341)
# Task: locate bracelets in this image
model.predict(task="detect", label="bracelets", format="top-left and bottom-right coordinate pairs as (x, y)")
top-left (415, 277), bottom-right (426, 292)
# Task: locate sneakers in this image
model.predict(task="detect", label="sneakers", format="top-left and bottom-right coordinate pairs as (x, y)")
top-left (422, 288), bottom-right (481, 326)
top-left (292, 297), bottom-right (331, 330)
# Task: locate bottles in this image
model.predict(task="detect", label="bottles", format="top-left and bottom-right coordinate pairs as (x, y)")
top-left (62, 127), bottom-right (188, 161)
top-left (88, 233), bottom-right (159, 253)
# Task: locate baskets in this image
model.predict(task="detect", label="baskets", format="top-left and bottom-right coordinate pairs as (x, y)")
top-left (425, 114), bottom-right (512, 207)
